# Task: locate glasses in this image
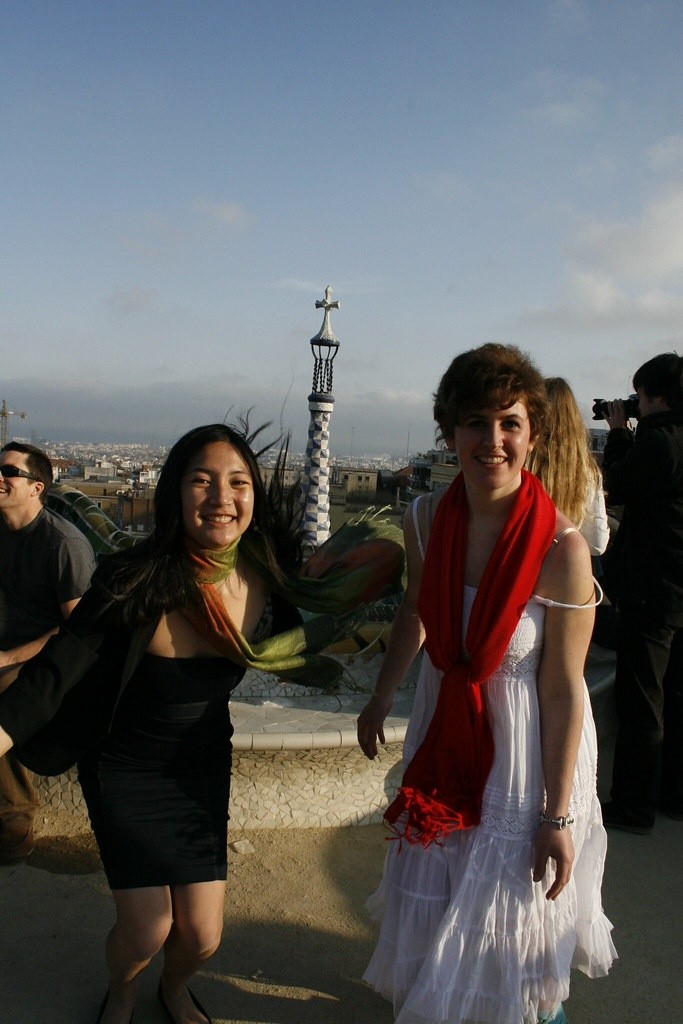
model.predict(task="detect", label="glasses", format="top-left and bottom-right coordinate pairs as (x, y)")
top-left (0, 464), bottom-right (42, 483)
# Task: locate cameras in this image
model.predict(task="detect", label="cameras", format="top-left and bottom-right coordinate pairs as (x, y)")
top-left (592, 394), bottom-right (641, 420)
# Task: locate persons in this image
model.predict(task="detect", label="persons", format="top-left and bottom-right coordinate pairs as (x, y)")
top-left (524, 377), bottom-right (610, 555)
top-left (0, 422), bottom-right (408, 1024)
top-left (355, 343), bottom-right (619, 1024)
top-left (0, 441), bottom-right (99, 865)
top-left (592, 353), bottom-right (683, 833)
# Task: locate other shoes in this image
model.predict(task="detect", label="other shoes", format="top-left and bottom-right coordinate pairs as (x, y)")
top-left (600, 800), bottom-right (655, 835)
top-left (155, 978), bottom-right (212, 1024)
top-left (96, 988), bottom-right (135, 1024)
top-left (0, 822), bottom-right (34, 861)
top-left (656, 794), bottom-right (683, 821)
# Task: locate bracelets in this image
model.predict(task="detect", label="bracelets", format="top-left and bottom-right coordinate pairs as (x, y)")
top-left (539, 812), bottom-right (574, 829)
